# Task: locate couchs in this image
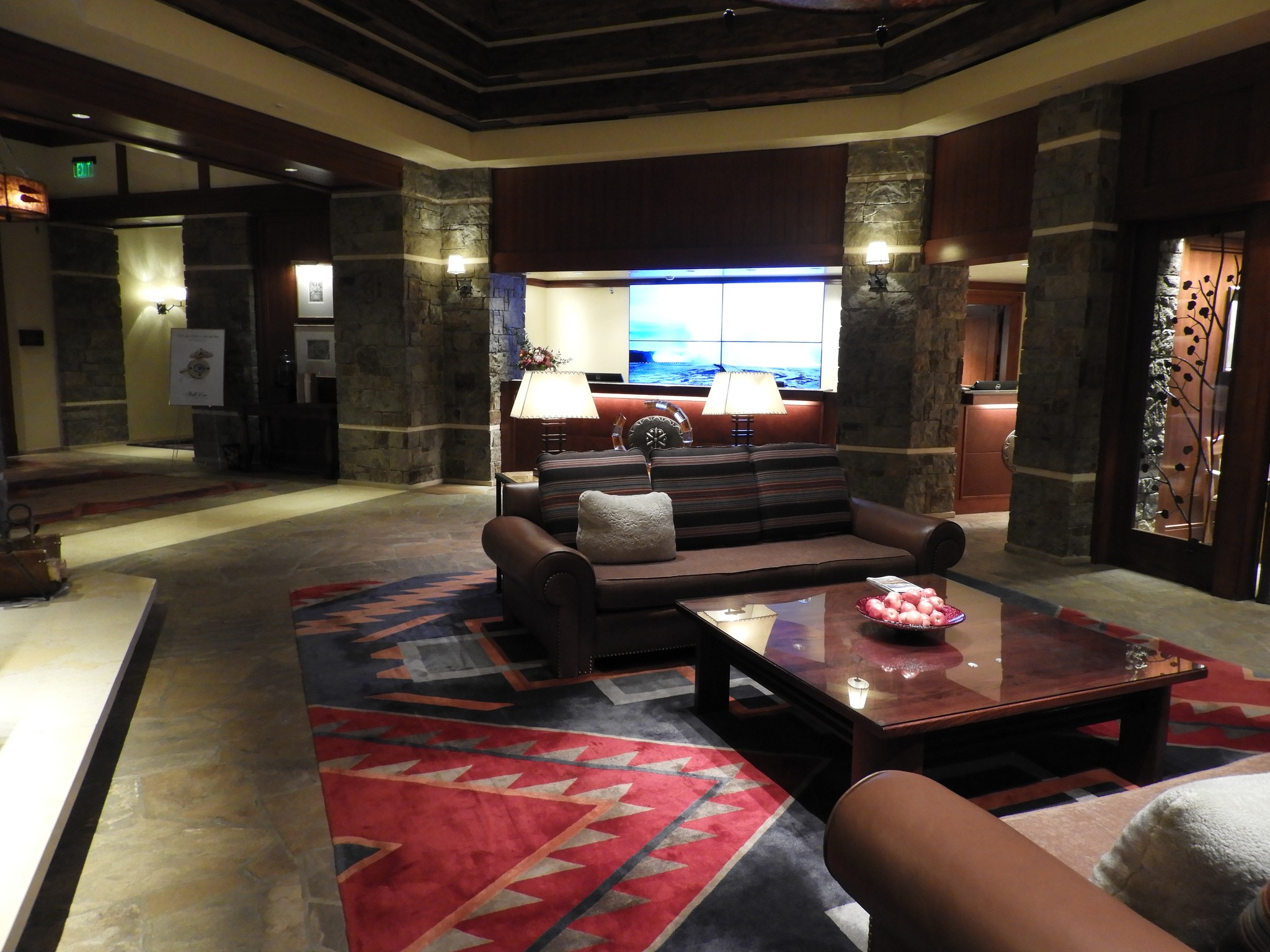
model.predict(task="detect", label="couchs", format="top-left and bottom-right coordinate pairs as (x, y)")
top-left (824, 751), bottom-right (1270, 952)
top-left (482, 483), bottom-right (966, 677)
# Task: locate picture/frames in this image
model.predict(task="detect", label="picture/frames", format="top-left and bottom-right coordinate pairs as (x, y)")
top-left (291, 260), bottom-right (336, 378)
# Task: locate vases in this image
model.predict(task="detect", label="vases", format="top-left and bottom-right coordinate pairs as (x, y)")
top-left (526, 364), bottom-right (546, 370)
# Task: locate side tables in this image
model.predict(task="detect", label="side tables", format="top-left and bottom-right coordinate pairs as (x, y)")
top-left (495, 471), bottom-right (539, 517)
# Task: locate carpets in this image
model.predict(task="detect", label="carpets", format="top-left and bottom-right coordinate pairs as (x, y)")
top-left (288, 572), bottom-right (1270, 952)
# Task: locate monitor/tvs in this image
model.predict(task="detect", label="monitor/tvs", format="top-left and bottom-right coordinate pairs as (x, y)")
top-left (627, 280), bottom-right (826, 389)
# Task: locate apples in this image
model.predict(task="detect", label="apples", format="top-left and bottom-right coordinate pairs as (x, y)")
top-left (866, 588), bottom-right (946, 626)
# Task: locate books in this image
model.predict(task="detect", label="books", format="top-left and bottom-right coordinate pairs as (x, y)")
top-left (866, 576), bottom-right (924, 594)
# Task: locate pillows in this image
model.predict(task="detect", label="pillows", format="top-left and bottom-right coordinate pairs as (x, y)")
top-left (1088, 773), bottom-right (1270, 952)
top-left (535, 443), bottom-right (853, 563)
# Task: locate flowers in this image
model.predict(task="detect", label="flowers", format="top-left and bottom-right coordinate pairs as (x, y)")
top-left (515, 331), bottom-right (572, 371)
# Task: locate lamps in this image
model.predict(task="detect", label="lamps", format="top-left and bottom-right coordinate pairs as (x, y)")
top-left (72, 156), bottom-right (97, 179)
top-left (702, 372), bottom-right (787, 446)
top-left (150, 287), bottom-right (187, 316)
top-left (447, 255), bottom-right (472, 294)
top-left (510, 371), bottom-right (600, 476)
top-left (866, 242), bottom-right (890, 288)
top-left (0, 136), bottom-right (49, 222)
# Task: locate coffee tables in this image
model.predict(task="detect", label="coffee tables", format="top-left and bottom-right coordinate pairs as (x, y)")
top-left (677, 574), bottom-right (1208, 787)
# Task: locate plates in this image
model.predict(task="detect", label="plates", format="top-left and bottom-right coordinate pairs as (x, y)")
top-left (854, 594), bottom-right (966, 631)
